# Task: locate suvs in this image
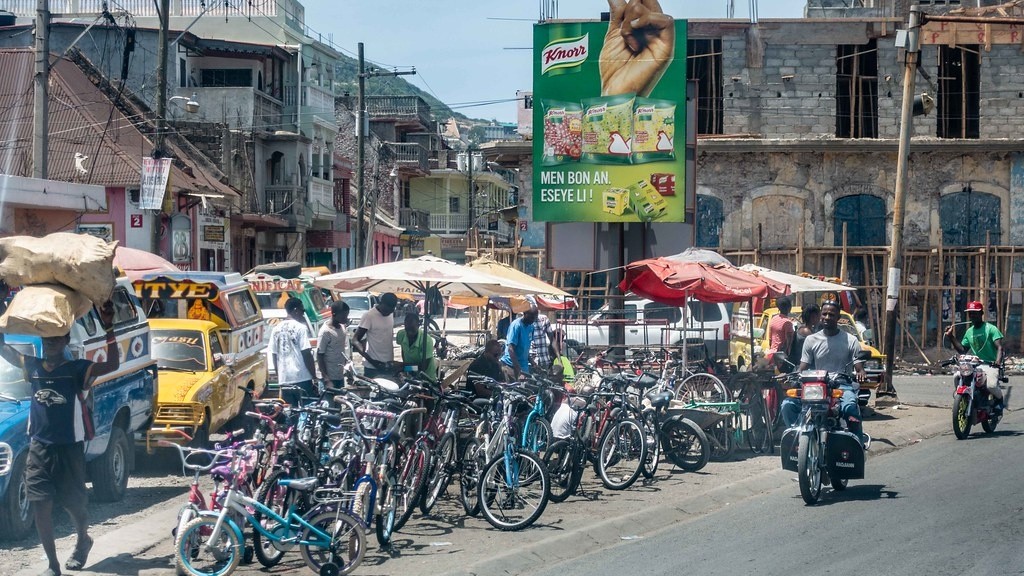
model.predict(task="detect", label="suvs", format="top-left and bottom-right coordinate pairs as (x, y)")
top-left (130, 269), bottom-right (270, 474)
top-left (730, 272), bottom-right (886, 389)
top-left (241, 261), bottom-right (339, 402)
top-left (551, 291), bottom-right (730, 345)
top-left (0, 275), bottom-right (158, 541)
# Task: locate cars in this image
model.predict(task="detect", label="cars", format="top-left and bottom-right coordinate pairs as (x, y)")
top-left (339, 290), bottom-right (378, 343)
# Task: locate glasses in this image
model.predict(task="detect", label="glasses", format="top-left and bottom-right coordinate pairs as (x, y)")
top-left (486, 351), bottom-right (503, 356)
top-left (298, 308), bottom-right (305, 312)
top-left (821, 301), bottom-right (841, 305)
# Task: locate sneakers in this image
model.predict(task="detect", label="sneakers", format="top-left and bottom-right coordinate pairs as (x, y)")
top-left (38, 565), bottom-right (61, 576)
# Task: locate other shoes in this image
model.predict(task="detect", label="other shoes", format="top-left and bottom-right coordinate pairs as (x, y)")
top-left (65, 537), bottom-right (94, 570)
top-left (46, 571), bottom-right (52, 572)
top-left (994, 402), bottom-right (1003, 414)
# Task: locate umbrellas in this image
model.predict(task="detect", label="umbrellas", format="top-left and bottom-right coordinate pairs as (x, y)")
top-left (450, 253), bottom-right (579, 330)
top-left (586, 247), bottom-right (791, 377)
top-left (306, 249), bottom-right (552, 432)
top-left (737, 264), bottom-right (857, 371)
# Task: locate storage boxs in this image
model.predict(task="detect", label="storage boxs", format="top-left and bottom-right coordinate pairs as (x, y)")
top-left (602, 173), bottom-right (675, 222)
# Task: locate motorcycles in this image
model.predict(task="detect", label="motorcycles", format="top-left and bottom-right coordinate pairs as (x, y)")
top-left (772, 350), bottom-right (872, 504)
top-left (937, 353), bottom-right (1009, 440)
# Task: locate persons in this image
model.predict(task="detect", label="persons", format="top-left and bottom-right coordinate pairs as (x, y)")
top-left (599, 0), bottom-right (675, 98)
top-left (944, 301), bottom-right (1004, 409)
top-left (777, 300), bottom-right (867, 439)
top-left (765, 297), bottom-right (794, 419)
top-left (0, 300), bottom-right (119, 576)
top-left (316, 301), bottom-right (350, 408)
top-left (497, 306), bottom-right (575, 387)
top-left (395, 314), bottom-right (436, 380)
top-left (270, 297), bottom-right (318, 426)
top-left (790, 303), bottom-right (821, 365)
top-left (551, 385), bottom-right (594, 444)
top-left (351, 293), bottom-right (397, 379)
top-left (466, 340), bottom-right (505, 398)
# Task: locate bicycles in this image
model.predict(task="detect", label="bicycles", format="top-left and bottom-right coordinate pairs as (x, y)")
top-left (174, 444), bottom-right (368, 576)
top-left (158, 342), bottom-right (784, 564)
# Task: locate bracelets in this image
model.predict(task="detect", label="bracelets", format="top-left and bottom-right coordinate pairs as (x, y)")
top-left (105, 325), bottom-right (114, 332)
top-left (106, 337), bottom-right (116, 344)
top-left (314, 377), bottom-right (318, 383)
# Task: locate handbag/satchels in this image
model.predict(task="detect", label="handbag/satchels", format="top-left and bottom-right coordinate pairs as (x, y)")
top-left (74, 358), bottom-right (94, 440)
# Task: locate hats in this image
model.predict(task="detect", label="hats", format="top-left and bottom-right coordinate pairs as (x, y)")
top-left (553, 328), bottom-right (566, 335)
top-left (964, 302), bottom-right (983, 312)
